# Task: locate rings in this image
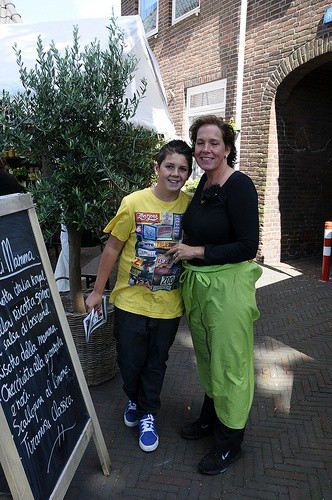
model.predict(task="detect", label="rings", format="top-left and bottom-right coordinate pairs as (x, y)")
top-left (173, 253), bottom-right (176, 257)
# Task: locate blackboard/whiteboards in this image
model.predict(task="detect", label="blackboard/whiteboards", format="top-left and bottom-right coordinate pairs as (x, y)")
top-left (1, 192), bottom-right (111, 500)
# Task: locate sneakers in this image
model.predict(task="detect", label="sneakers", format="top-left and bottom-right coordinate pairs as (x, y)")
top-left (124, 399), bottom-right (140, 427)
top-left (138, 411), bottom-right (160, 452)
top-left (180, 418), bottom-right (221, 440)
top-left (198, 443), bottom-right (242, 475)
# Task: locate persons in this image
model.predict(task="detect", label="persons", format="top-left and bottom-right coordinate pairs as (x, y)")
top-left (164, 115), bottom-right (263, 476)
top-left (85, 140), bottom-right (193, 452)
